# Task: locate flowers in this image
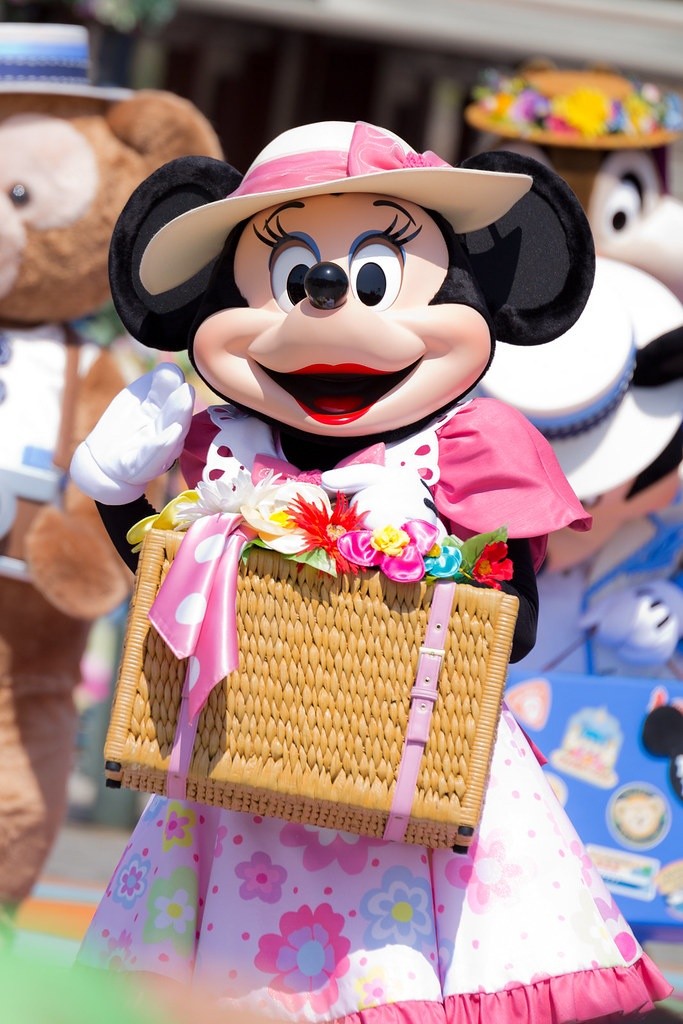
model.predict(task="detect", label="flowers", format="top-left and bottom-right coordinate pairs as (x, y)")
top-left (128, 476), bottom-right (513, 590)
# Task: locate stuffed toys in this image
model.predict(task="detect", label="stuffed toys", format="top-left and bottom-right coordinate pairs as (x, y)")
top-left (74, 118), bottom-right (682, 1021)
top-left (1, 22), bottom-right (225, 942)
top-left (472, 71), bottom-right (683, 312)
